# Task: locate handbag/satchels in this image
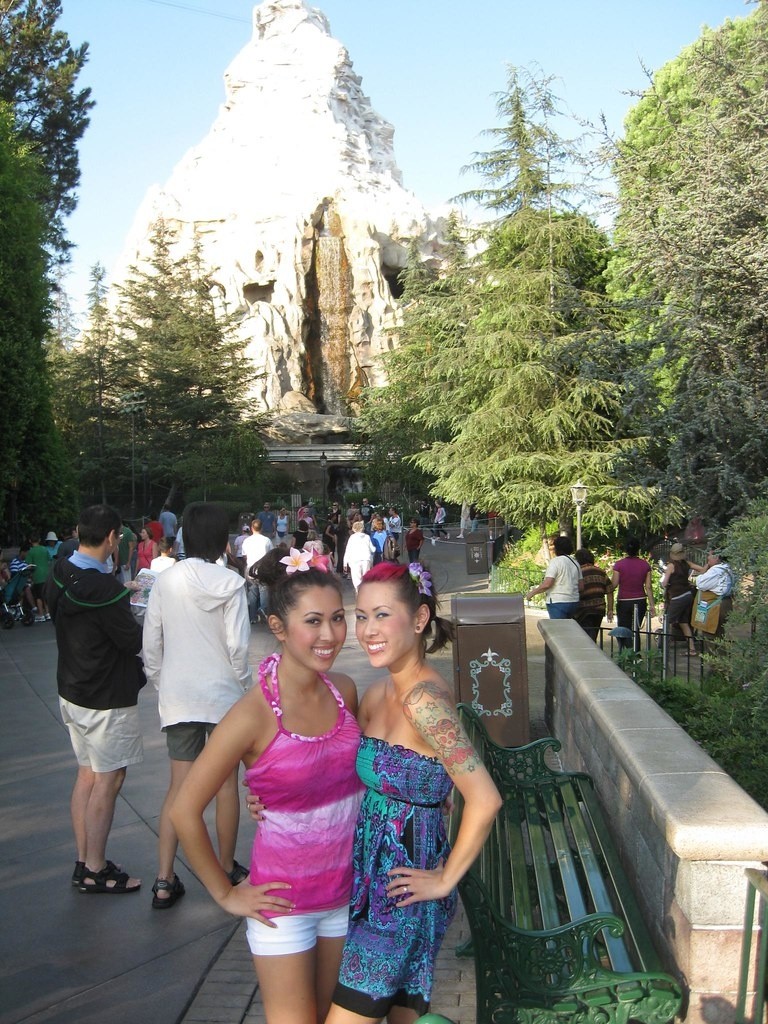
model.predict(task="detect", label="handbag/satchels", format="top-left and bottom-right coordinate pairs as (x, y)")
top-left (690, 591), bottom-right (723, 634)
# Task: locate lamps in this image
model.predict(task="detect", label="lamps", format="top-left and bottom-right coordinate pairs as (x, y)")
top-left (607, 626), bottom-right (634, 668)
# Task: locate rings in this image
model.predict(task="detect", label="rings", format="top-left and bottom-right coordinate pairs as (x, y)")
top-left (403, 886), bottom-right (408, 894)
top-left (246, 803), bottom-right (250, 809)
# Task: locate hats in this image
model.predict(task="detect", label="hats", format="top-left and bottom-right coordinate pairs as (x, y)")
top-left (243, 526), bottom-right (250, 531)
top-left (670, 543), bottom-right (686, 561)
top-left (45, 532), bottom-right (58, 541)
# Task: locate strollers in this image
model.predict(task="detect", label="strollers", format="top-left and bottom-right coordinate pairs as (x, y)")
top-left (0, 563), bottom-right (38, 629)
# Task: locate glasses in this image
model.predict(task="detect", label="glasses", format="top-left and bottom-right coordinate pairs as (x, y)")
top-left (410, 522), bottom-right (416, 523)
top-left (264, 506), bottom-right (269, 508)
top-left (303, 512), bottom-right (308, 513)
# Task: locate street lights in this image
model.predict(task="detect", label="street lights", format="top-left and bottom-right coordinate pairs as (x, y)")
top-left (319, 451), bottom-right (328, 507)
top-left (116, 390), bottom-right (145, 518)
top-left (569, 478), bottom-right (588, 550)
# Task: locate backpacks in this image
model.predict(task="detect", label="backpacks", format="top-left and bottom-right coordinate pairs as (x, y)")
top-left (384, 530), bottom-right (401, 561)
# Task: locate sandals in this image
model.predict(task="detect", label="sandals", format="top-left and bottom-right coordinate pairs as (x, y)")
top-left (224, 859), bottom-right (250, 886)
top-left (680, 649), bottom-right (696, 657)
top-left (152, 873), bottom-right (185, 909)
top-left (72, 860), bottom-right (141, 894)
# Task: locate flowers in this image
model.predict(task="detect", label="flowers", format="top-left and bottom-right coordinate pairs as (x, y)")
top-left (409, 557), bottom-right (437, 598)
top-left (277, 538), bottom-right (336, 576)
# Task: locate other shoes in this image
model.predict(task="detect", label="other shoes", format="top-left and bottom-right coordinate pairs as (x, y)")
top-left (251, 607), bottom-right (268, 623)
top-left (342, 572), bottom-right (351, 580)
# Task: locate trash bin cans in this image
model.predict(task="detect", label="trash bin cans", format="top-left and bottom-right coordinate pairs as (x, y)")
top-left (452, 594), bottom-right (531, 749)
top-left (239, 512), bottom-right (255, 535)
top-left (466, 531), bottom-right (488, 576)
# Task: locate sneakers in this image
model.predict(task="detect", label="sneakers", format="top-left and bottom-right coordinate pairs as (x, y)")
top-left (447, 533), bottom-right (451, 539)
top-left (457, 534), bottom-right (464, 539)
top-left (32, 607), bottom-right (51, 622)
top-left (437, 537), bottom-right (441, 540)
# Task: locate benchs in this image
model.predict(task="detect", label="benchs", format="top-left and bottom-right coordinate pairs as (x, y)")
top-left (443, 693), bottom-right (686, 1024)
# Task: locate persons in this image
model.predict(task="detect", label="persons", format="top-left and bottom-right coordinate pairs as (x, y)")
top-left (0, 504), bottom-right (186, 624)
top-left (489, 511), bottom-right (502, 541)
top-left (168, 561), bottom-right (503, 1024)
top-left (611, 534), bottom-right (655, 665)
top-left (256, 502), bottom-right (289, 547)
top-left (216, 519), bottom-right (275, 623)
top-left (416, 499), bottom-right (450, 540)
top-left (291, 500), bottom-right (318, 550)
top-left (47, 505), bottom-right (147, 893)
top-left (456, 501), bottom-right (479, 539)
top-left (343, 521), bottom-right (394, 597)
top-left (322, 500), bottom-right (349, 575)
top-left (345, 498), bottom-right (401, 542)
top-left (662, 543), bottom-right (733, 657)
top-left (574, 549), bottom-right (613, 643)
top-left (405, 517), bottom-right (423, 563)
top-left (526, 536), bottom-right (584, 619)
top-left (143, 507), bottom-right (250, 909)
